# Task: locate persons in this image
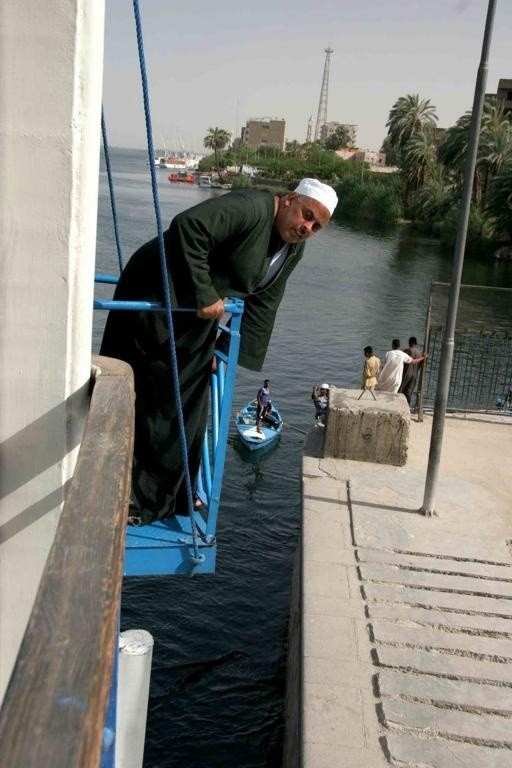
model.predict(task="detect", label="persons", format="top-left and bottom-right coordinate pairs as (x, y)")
top-left (97, 176), bottom-right (342, 529)
top-left (360, 345), bottom-right (381, 391)
top-left (310, 382), bottom-right (330, 418)
top-left (256, 378), bottom-right (271, 435)
top-left (397, 336), bottom-right (422, 405)
top-left (263, 400), bottom-right (283, 430)
top-left (377, 338), bottom-right (429, 393)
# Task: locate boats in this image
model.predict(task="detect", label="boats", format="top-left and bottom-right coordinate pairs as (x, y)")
top-left (169, 167), bottom-right (234, 191)
top-left (231, 437), bottom-right (282, 465)
top-left (234, 392), bottom-right (287, 454)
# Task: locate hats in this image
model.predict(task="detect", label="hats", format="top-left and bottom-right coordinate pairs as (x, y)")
top-left (320, 383), bottom-right (330, 389)
top-left (293, 177), bottom-right (338, 218)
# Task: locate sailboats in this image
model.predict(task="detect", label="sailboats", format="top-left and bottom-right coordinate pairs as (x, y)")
top-left (147, 127), bottom-right (209, 171)
